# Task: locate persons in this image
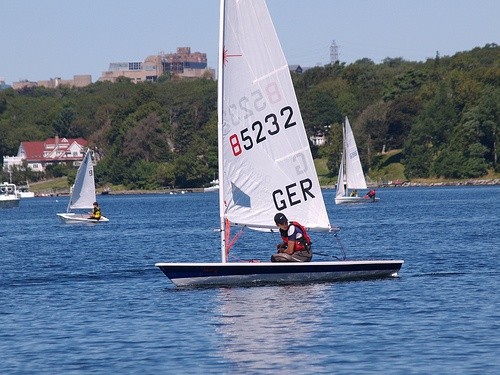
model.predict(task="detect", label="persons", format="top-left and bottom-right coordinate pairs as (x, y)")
top-left (271, 213), bottom-right (313, 262)
top-left (88, 202), bottom-right (101, 221)
top-left (350, 189), bottom-right (375, 200)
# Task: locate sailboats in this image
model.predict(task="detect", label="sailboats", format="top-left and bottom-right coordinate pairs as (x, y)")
top-left (155, 0), bottom-right (405, 287)
top-left (56, 148), bottom-right (109, 223)
top-left (331, 116), bottom-right (385, 204)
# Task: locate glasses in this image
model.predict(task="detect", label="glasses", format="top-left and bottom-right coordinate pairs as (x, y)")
top-left (277, 221), bottom-right (288, 226)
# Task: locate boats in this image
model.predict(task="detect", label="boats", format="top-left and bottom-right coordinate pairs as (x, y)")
top-left (0, 183), bottom-right (22, 202)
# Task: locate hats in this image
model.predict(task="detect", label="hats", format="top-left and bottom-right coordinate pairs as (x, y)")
top-left (274, 213), bottom-right (289, 223)
